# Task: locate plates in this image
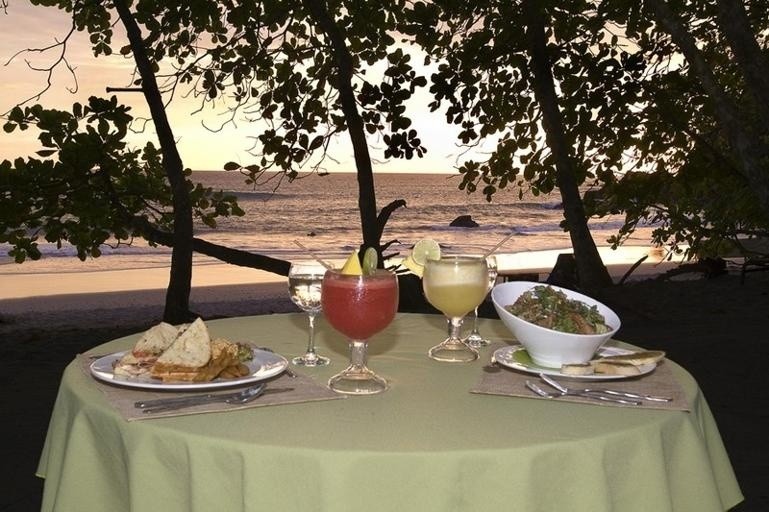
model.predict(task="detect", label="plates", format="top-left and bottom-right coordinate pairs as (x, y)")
top-left (89, 345), bottom-right (289, 393)
top-left (493, 343), bottom-right (657, 383)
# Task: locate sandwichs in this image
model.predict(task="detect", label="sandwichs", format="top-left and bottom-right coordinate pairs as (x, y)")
top-left (119, 317), bottom-right (212, 379)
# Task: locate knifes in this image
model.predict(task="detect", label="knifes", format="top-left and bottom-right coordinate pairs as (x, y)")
top-left (538, 372), bottom-right (675, 402)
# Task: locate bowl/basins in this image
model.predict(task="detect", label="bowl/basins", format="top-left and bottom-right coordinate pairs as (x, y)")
top-left (491, 280), bottom-right (622, 369)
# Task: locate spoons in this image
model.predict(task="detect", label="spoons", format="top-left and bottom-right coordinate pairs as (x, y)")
top-left (135, 382), bottom-right (267, 409)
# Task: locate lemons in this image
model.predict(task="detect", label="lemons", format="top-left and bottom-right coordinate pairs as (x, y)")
top-left (412, 237), bottom-right (441, 266)
top-left (363, 247), bottom-right (378, 274)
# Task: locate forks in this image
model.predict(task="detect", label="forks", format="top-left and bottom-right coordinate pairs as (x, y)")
top-left (524, 379), bottom-right (643, 406)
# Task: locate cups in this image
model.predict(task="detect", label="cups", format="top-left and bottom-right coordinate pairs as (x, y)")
top-left (423, 257), bottom-right (488, 363)
top-left (287, 262), bottom-right (336, 366)
top-left (461, 256), bottom-right (500, 347)
top-left (322, 268), bottom-right (399, 396)
